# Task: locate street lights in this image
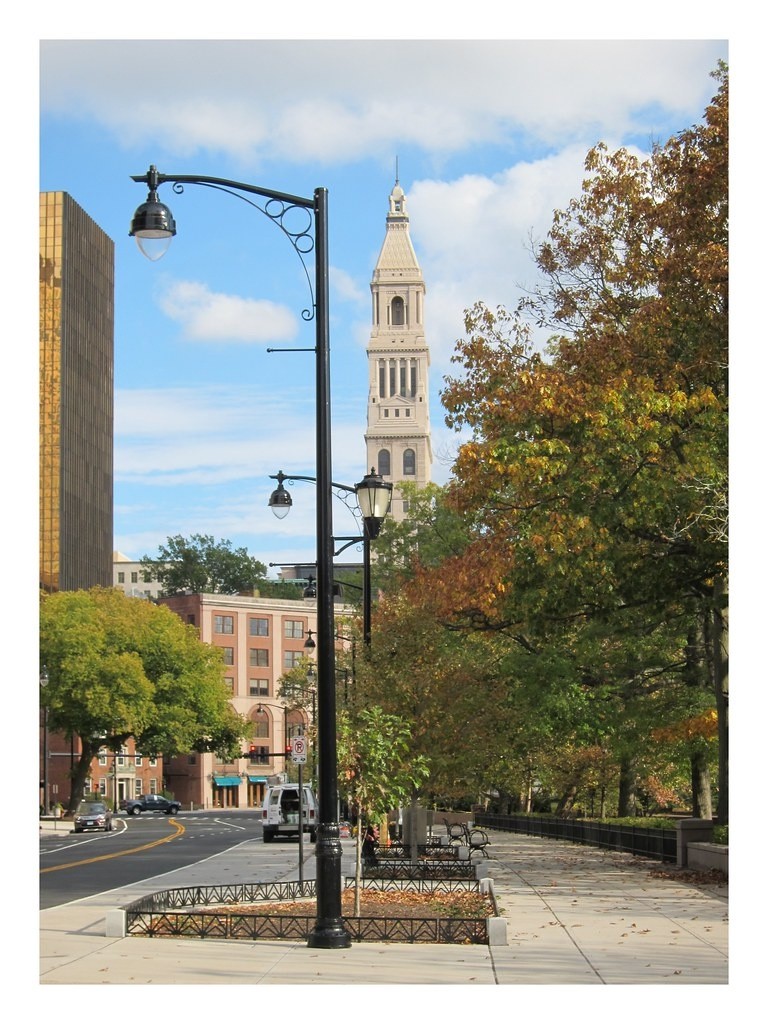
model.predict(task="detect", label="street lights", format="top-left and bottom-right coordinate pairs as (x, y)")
top-left (40, 664), bottom-right (50, 816)
top-left (256, 702), bottom-right (288, 770)
top-left (129, 165), bottom-right (393, 950)
top-left (280, 685), bottom-right (316, 775)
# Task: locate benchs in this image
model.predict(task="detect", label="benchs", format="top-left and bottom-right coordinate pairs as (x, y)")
top-left (461, 822), bottom-right (491, 860)
top-left (442, 817), bottom-right (466, 849)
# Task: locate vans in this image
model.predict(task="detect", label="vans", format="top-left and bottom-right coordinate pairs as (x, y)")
top-left (262, 783), bottom-right (317, 843)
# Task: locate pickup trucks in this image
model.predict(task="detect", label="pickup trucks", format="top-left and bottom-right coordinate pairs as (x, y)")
top-left (120, 795), bottom-right (182, 816)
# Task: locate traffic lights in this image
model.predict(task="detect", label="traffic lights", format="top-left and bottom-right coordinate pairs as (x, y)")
top-left (286, 746), bottom-right (291, 752)
top-left (250, 746), bottom-right (256, 752)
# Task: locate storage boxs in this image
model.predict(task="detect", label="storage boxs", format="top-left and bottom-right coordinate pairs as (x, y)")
top-left (303, 833), bottom-right (311, 843)
top-left (287, 814), bottom-right (299, 824)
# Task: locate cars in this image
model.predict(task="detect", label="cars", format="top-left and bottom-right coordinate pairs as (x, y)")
top-left (74, 799), bottom-right (112, 832)
